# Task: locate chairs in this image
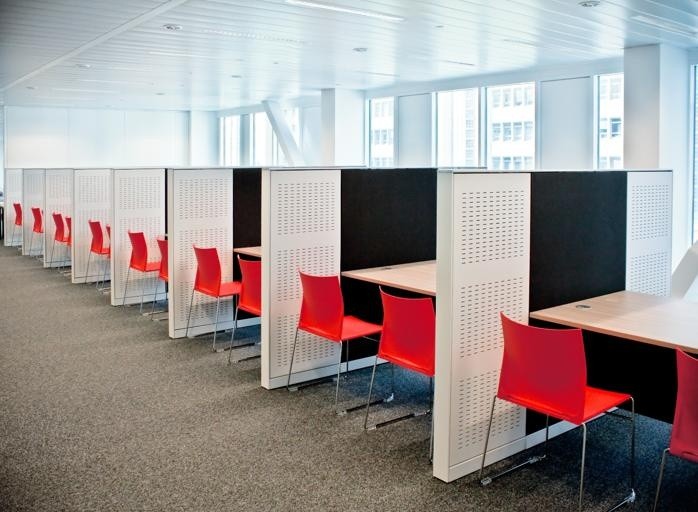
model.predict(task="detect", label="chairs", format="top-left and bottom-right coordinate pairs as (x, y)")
top-left (286, 268), bottom-right (434, 464)
top-left (122, 228), bottom-right (168, 322)
top-left (184, 243), bottom-right (261, 372)
top-left (11, 202), bottom-right (110, 296)
top-left (655, 348), bottom-right (698, 512)
top-left (481, 313), bottom-right (638, 511)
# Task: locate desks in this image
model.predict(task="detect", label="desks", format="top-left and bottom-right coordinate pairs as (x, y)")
top-left (530, 289), bottom-right (697, 449)
top-left (342, 257), bottom-right (437, 373)
